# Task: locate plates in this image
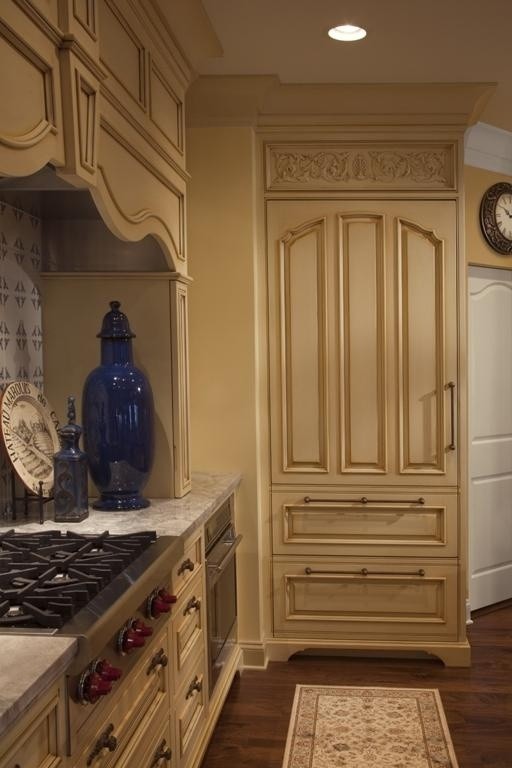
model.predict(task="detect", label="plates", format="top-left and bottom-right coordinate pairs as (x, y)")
top-left (0, 381), bottom-right (62, 500)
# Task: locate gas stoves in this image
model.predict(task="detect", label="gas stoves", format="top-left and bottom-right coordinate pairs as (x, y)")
top-left (0, 528), bottom-right (182, 753)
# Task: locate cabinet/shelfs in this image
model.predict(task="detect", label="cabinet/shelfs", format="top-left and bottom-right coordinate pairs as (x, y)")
top-left (38, 270), bottom-right (192, 501)
top-left (265, 199), bottom-right (470, 669)
top-left (0, 492), bottom-right (245, 768)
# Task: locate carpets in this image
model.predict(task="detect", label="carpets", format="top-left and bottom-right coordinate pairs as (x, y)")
top-left (282, 684), bottom-right (459, 768)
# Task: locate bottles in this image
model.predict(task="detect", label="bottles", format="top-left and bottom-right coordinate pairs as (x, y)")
top-left (51, 413), bottom-right (89, 523)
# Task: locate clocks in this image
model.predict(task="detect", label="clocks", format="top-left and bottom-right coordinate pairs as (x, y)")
top-left (480, 182), bottom-right (512, 255)
